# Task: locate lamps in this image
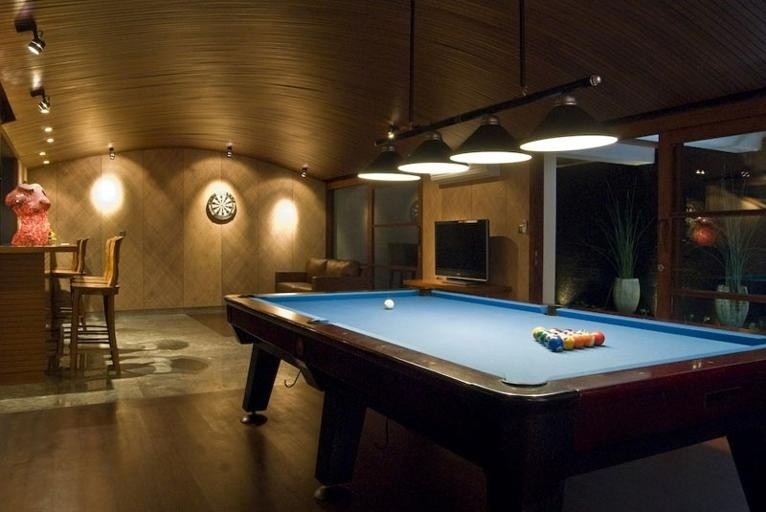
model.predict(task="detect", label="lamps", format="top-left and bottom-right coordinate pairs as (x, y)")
top-left (225, 143), bottom-right (233, 158)
top-left (355, 0), bottom-right (621, 182)
top-left (109, 147), bottom-right (117, 160)
top-left (299, 165), bottom-right (309, 179)
top-left (30, 84), bottom-right (53, 113)
top-left (13, 18), bottom-right (47, 57)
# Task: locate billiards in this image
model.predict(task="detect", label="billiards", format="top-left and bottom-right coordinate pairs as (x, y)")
top-left (532, 326), bottom-right (605, 351)
top-left (384, 299), bottom-right (394, 309)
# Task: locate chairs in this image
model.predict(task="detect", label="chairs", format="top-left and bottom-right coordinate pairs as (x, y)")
top-left (41, 231), bottom-right (127, 381)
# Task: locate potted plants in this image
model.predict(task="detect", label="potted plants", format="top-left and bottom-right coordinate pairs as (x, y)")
top-left (693, 183), bottom-right (765, 328)
top-left (586, 180), bottom-right (650, 311)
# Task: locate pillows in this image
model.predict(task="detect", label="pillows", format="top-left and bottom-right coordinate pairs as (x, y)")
top-left (322, 257), bottom-right (357, 277)
top-left (302, 256), bottom-right (326, 280)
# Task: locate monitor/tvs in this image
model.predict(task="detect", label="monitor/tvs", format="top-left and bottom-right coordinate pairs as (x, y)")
top-left (434, 219), bottom-right (489, 285)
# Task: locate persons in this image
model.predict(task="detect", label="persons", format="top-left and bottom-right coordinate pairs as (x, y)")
top-left (5, 183), bottom-right (52, 245)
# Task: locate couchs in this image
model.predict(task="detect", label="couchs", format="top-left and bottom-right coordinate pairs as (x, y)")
top-left (271, 259), bottom-right (370, 295)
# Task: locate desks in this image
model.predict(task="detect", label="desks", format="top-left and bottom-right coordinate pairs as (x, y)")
top-left (218, 286), bottom-right (766, 511)
